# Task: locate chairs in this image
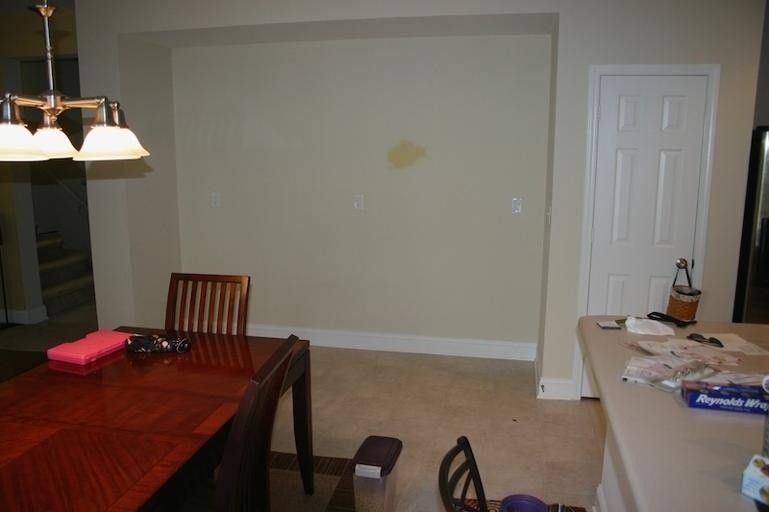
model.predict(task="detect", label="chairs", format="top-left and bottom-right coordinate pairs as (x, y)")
top-left (439, 436), bottom-right (491, 511)
top-left (166, 272), bottom-right (297, 511)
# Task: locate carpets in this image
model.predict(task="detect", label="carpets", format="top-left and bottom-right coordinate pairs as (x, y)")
top-left (264, 446), bottom-right (355, 510)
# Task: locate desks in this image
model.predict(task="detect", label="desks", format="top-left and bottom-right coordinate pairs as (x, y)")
top-left (576, 312), bottom-right (769, 511)
top-left (1, 326), bottom-right (315, 512)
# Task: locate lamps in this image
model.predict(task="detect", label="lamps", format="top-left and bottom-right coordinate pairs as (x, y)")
top-left (0, 0), bottom-right (150, 161)
